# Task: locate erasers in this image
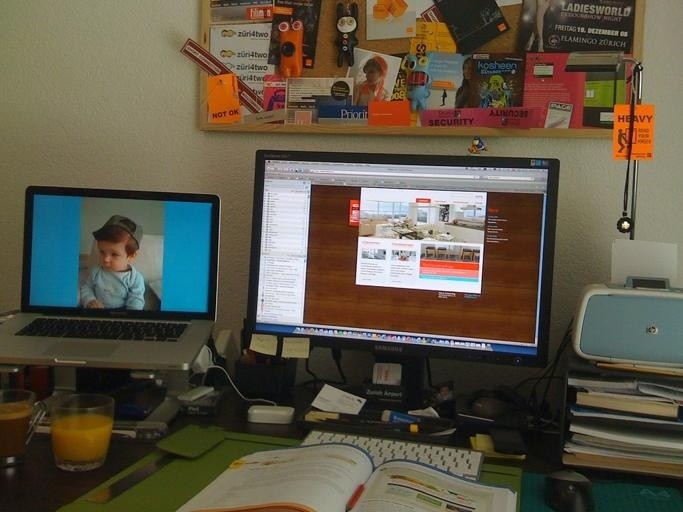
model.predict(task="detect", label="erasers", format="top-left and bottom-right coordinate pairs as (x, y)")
top-left (380, 409), bottom-right (420, 423)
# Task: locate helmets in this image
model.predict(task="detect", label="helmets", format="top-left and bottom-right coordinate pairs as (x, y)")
top-left (373, 55), bottom-right (389, 75)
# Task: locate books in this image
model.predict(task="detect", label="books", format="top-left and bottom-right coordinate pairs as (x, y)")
top-left (563, 360), bottom-right (683, 479)
top-left (173, 441), bottom-right (519, 512)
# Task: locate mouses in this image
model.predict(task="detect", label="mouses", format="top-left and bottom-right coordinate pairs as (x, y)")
top-left (545, 468), bottom-right (595, 512)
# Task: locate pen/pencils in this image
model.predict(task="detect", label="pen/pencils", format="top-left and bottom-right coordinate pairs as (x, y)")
top-left (358, 421), bottom-right (419, 433)
top-left (346, 484), bottom-right (364, 512)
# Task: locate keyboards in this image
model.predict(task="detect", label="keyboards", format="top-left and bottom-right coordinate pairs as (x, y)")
top-left (298, 428), bottom-right (485, 482)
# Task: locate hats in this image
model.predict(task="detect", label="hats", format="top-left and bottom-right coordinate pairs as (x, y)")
top-left (102, 214), bottom-right (144, 249)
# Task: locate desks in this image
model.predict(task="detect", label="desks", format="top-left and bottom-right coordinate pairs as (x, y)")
top-left (0, 383), bottom-right (679, 512)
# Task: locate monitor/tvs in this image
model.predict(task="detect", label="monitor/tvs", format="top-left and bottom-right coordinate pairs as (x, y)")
top-left (244, 149), bottom-right (561, 454)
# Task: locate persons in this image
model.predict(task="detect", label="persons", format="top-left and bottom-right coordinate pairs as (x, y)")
top-left (523, 2), bottom-right (550, 56)
top-left (352, 56), bottom-right (389, 107)
top-left (440, 88), bottom-right (448, 106)
top-left (437, 385), bottom-right (455, 402)
top-left (453, 57), bottom-right (480, 108)
top-left (77, 214), bottom-right (146, 309)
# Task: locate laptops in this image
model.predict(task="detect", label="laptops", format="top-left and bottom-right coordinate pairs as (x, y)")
top-left (0, 185), bottom-right (223, 371)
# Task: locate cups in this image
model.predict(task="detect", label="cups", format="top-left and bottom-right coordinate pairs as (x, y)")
top-left (48, 391), bottom-right (116, 474)
top-left (0, 387), bottom-right (38, 469)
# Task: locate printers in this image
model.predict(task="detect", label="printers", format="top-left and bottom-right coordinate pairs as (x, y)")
top-left (570, 275), bottom-right (683, 370)
